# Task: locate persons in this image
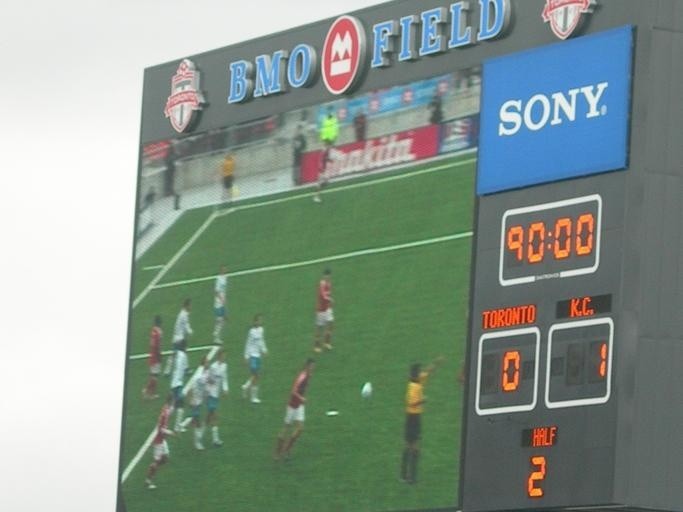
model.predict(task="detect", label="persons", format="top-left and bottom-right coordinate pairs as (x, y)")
top-left (272, 358), bottom-right (314, 461)
top-left (222, 151), bottom-right (235, 201)
top-left (164, 297), bottom-right (193, 377)
top-left (240, 313), bottom-right (268, 403)
top-left (142, 314), bottom-right (163, 399)
top-left (427, 89), bottom-right (444, 124)
top-left (398, 357), bottom-right (444, 485)
top-left (144, 341), bottom-right (230, 491)
top-left (143, 186), bottom-right (157, 210)
top-left (313, 269), bottom-right (335, 353)
top-left (212, 265), bottom-right (227, 344)
top-left (293, 111), bottom-right (366, 160)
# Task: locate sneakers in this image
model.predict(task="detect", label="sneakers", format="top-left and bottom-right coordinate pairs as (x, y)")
top-left (170, 425), bottom-right (225, 451)
top-left (313, 347), bottom-right (320, 352)
top-left (240, 385), bottom-right (247, 399)
top-left (142, 389), bottom-right (158, 402)
top-left (250, 398), bottom-right (261, 403)
top-left (144, 477), bottom-right (155, 489)
top-left (322, 342), bottom-right (333, 351)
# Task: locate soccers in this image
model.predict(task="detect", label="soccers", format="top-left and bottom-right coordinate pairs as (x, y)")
top-left (361, 381), bottom-right (372, 397)
